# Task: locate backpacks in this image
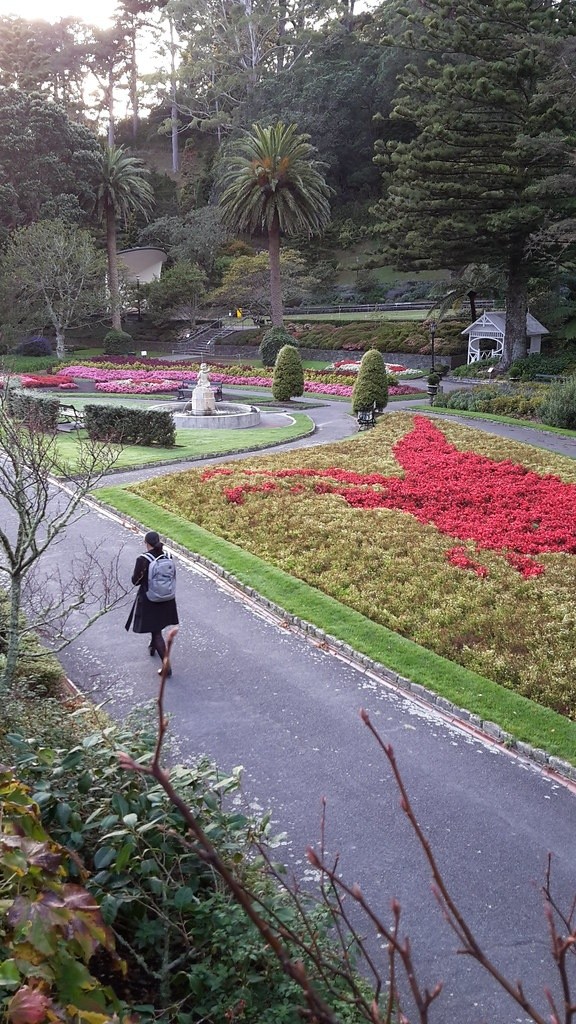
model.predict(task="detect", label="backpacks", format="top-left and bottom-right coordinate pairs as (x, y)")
top-left (141, 550), bottom-right (176, 602)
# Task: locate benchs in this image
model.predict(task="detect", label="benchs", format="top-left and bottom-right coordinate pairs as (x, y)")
top-left (59, 403), bottom-right (86, 430)
top-left (357, 398), bottom-right (379, 430)
top-left (176, 380), bottom-right (223, 402)
top-left (64, 346), bottom-right (80, 356)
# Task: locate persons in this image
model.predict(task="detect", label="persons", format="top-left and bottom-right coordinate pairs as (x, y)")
top-left (125, 532), bottom-right (179, 679)
top-left (197, 362), bottom-right (212, 387)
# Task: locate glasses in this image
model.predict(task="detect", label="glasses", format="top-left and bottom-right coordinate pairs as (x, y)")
top-left (144, 541), bottom-right (147, 544)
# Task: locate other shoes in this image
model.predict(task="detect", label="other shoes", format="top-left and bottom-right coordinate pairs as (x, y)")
top-left (157, 668), bottom-right (172, 676)
top-left (148, 645), bottom-right (155, 655)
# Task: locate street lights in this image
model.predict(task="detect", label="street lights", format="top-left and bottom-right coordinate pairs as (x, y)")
top-left (135, 274), bottom-right (142, 322)
top-left (429, 317), bottom-right (437, 374)
